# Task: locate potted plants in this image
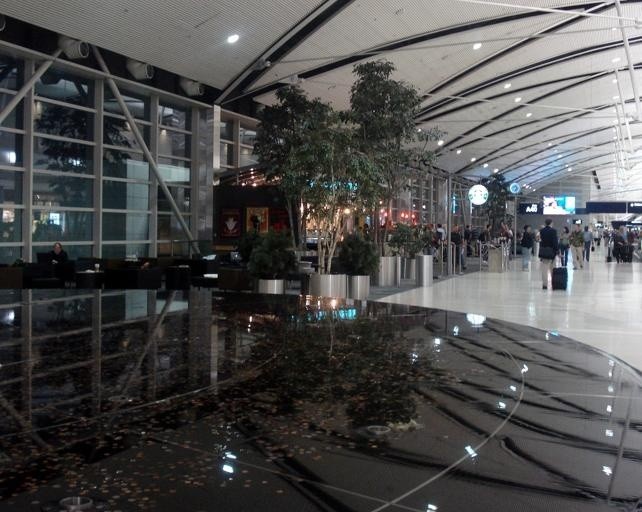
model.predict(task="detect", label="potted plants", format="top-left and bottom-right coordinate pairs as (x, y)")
top-left (478, 171), bottom-right (514, 272)
top-left (236, 51), bottom-right (449, 295)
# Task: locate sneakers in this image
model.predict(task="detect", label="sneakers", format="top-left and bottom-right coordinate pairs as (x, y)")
top-left (583, 256), bottom-right (589, 261)
top-left (573, 265), bottom-right (583, 270)
top-left (542, 285), bottom-right (547, 289)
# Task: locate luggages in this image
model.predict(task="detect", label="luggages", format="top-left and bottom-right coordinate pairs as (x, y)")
top-left (623, 245), bottom-right (634, 262)
top-left (552, 265), bottom-right (567, 290)
top-left (607, 256), bottom-right (612, 262)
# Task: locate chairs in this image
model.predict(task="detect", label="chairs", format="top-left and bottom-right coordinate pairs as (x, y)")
top-left (20, 253), bottom-right (252, 293)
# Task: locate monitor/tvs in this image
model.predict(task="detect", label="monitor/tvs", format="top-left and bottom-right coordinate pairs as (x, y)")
top-left (573, 219), bottom-right (582, 224)
top-left (543, 196), bottom-right (575, 215)
top-left (519, 203), bottom-right (539, 214)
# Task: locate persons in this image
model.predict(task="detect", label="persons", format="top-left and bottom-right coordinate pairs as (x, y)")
top-left (448, 224), bottom-right (466, 275)
top-left (462, 220), bottom-right (642, 273)
top-left (48, 243), bottom-right (69, 264)
top-left (537, 218), bottom-right (560, 290)
top-left (430, 223), bottom-right (446, 264)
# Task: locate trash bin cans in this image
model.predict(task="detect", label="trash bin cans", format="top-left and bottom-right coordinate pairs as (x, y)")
top-left (416, 254), bottom-right (433, 286)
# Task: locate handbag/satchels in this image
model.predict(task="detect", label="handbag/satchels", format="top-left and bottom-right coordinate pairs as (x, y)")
top-left (539, 247), bottom-right (555, 259)
top-left (591, 246), bottom-right (595, 252)
